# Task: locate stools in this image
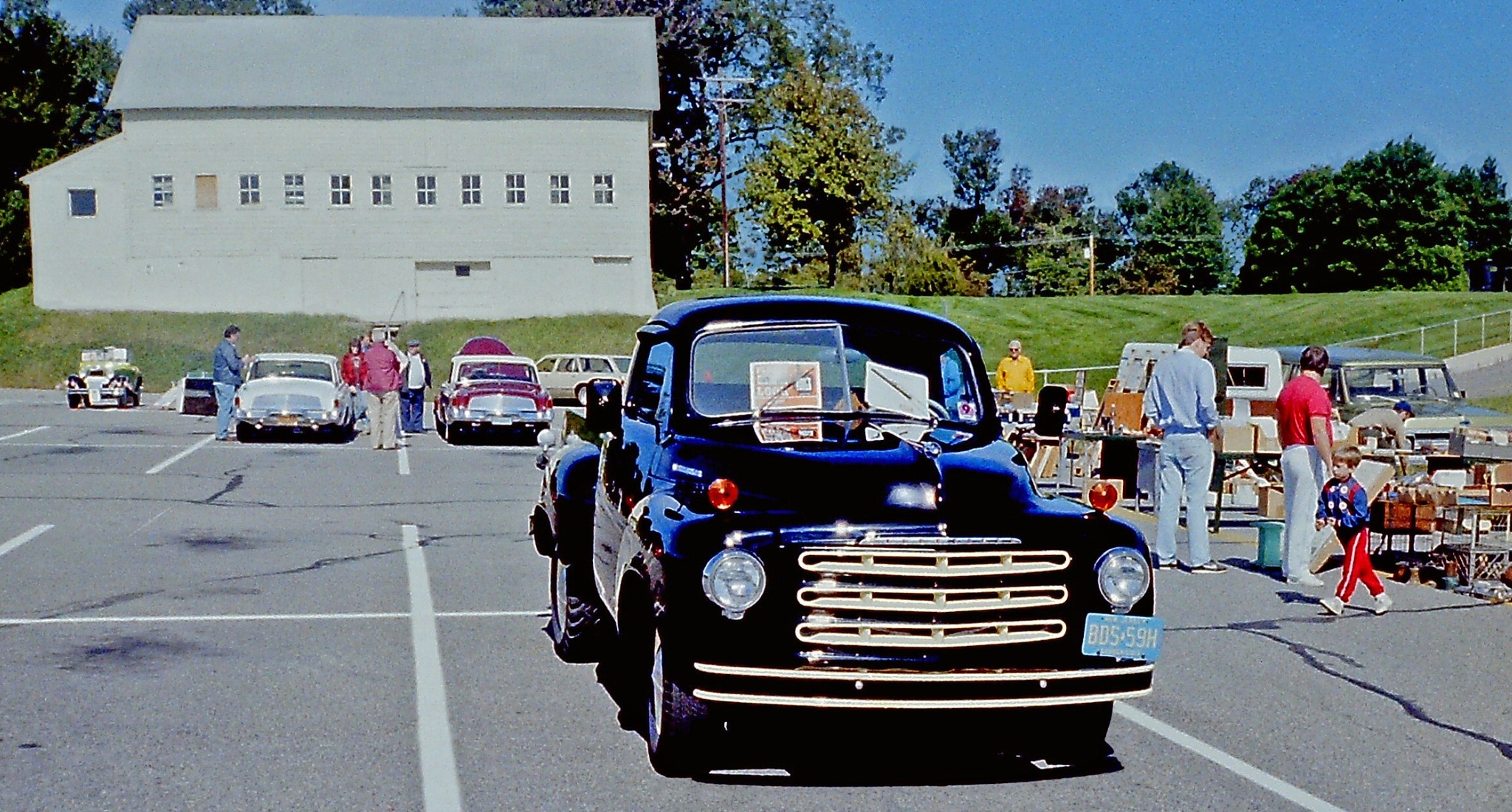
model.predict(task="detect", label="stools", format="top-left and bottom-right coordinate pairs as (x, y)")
top-left (1441, 463), bottom-right (1512, 582)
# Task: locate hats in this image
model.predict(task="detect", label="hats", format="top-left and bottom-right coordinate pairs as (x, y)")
top-left (406, 340), bottom-right (419, 346)
top-left (1009, 340), bottom-right (1022, 352)
top-left (1395, 400), bottom-right (1415, 417)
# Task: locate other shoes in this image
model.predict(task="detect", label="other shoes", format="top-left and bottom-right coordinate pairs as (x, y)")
top-left (415, 429), bottom-right (427, 434)
top-left (384, 444), bottom-right (401, 450)
top-left (1287, 575), bottom-right (1324, 587)
top-left (216, 436), bottom-right (235, 442)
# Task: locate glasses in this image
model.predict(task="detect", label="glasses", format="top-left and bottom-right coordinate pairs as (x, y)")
top-left (1010, 348), bottom-right (1018, 352)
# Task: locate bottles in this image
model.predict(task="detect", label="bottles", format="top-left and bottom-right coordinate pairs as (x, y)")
top-left (1109, 403), bottom-right (1118, 435)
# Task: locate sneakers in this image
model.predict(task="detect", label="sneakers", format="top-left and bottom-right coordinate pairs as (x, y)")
top-left (1191, 560), bottom-right (1227, 573)
top-left (1375, 594), bottom-right (1393, 614)
top-left (1320, 596), bottom-right (1344, 614)
top-left (1159, 559), bottom-right (1185, 570)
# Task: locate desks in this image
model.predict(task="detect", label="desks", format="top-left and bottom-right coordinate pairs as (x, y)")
top-left (1059, 428), bottom-right (1149, 498)
top-left (1182, 452), bottom-right (1498, 533)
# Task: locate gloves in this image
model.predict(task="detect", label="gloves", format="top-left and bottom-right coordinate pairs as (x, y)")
top-left (350, 385), bottom-right (357, 394)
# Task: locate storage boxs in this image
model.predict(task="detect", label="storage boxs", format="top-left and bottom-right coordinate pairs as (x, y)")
top-left (994, 391), bottom-right (1512, 571)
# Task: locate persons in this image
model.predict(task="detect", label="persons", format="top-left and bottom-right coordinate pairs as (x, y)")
top-left (214, 325), bottom-right (255, 440)
top-left (1145, 322), bottom-right (1227, 574)
top-left (996, 340), bottom-right (1035, 407)
top-left (1347, 401), bottom-right (1415, 449)
top-left (1276, 346), bottom-right (1335, 587)
top-left (1316, 446), bottom-right (1392, 616)
top-left (341, 329), bottom-right (432, 450)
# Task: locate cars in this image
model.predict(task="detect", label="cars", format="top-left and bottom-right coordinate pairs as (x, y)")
top-left (1275, 345), bottom-right (1512, 452)
top-left (66, 348), bottom-right (142, 410)
top-left (232, 352), bottom-right (357, 444)
top-left (533, 353), bottom-right (632, 408)
top-left (432, 355), bottom-right (554, 444)
top-left (527, 295), bottom-right (1164, 777)
